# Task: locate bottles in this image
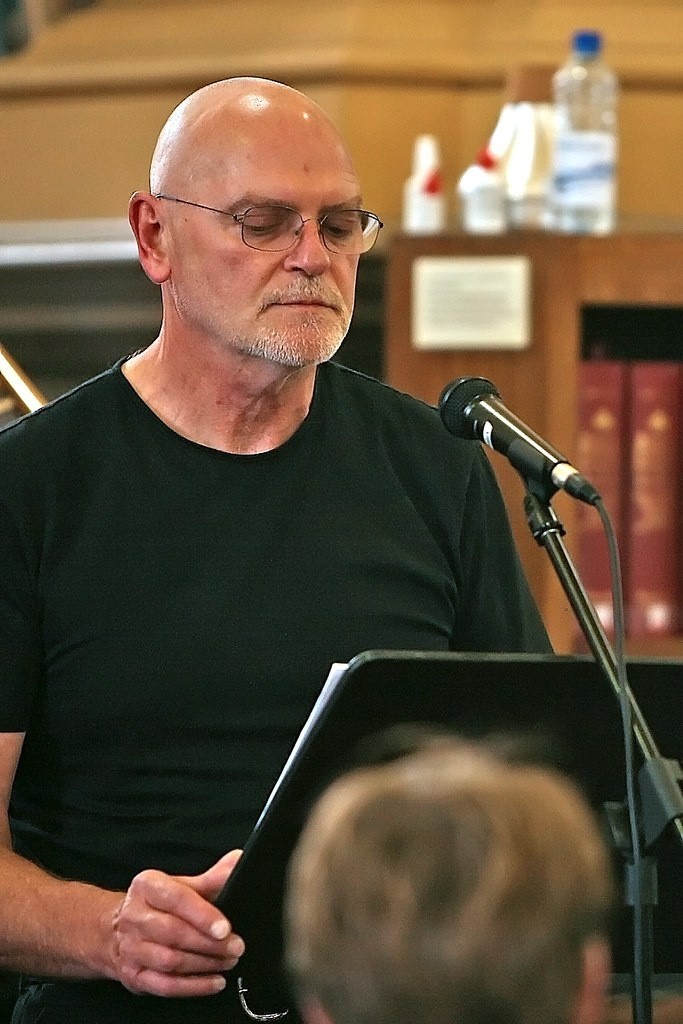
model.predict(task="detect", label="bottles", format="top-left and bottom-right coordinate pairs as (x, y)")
top-left (534, 28), bottom-right (621, 236)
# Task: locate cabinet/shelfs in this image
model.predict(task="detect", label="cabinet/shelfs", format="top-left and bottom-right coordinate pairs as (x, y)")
top-left (381, 233), bottom-right (682, 659)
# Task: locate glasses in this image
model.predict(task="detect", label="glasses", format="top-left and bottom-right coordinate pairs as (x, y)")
top-left (154, 195), bottom-right (385, 256)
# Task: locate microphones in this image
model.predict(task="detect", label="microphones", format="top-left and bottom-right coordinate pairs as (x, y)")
top-left (437, 376), bottom-right (602, 506)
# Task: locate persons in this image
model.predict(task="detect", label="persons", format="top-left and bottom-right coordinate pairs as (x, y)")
top-left (284, 752), bottom-right (613, 1024)
top-left (0, 77), bottom-right (554, 1024)
